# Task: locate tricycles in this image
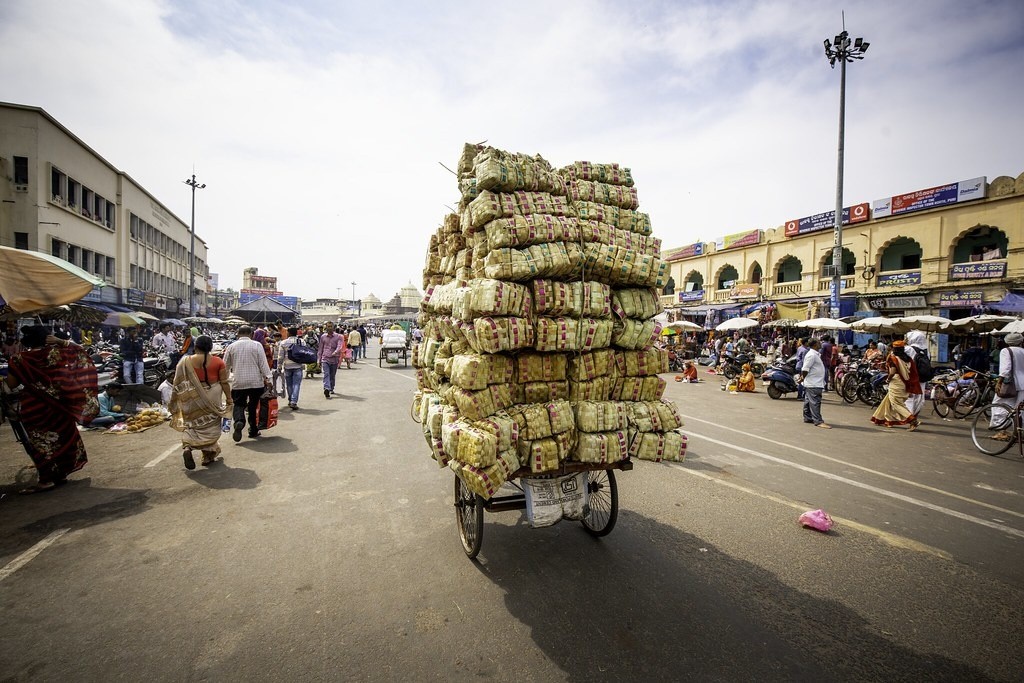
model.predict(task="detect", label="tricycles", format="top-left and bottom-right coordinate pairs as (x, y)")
top-left (454, 459), bottom-right (635, 560)
top-left (378, 348), bottom-right (408, 368)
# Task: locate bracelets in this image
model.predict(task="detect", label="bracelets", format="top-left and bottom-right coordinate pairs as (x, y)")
top-left (64, 340), bottom-right (69, 346)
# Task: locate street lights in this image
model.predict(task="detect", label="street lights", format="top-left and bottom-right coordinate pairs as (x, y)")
top-left (351, 281), bottom-right (357, 327)
top-left (824, 10), bottom-right (870, 348)
top-left (186, 175), bottom-right (205, 317)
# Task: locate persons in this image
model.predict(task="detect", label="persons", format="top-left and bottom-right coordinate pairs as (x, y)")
top-left (669, 332), bottom-right (1024, 441)
top-left (2, 319), bottom-right (425, 492)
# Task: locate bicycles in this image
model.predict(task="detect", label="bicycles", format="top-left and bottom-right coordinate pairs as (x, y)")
top-left (834, 358), bottom-right (868, 404)
top-left (929, 365), bottom-right (1024, 459)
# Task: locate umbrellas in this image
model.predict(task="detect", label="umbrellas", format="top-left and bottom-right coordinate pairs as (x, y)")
top-left (129, 310), bottom-right (160, 322)
top-left (162, 318), bottom-right (188, 326)
top-left (0, 244), bottom-right (107, 315)
top-left (667, 321), bottom-right (701, 332)
top-left (975, 293), bottom-right (1024, 313)
top-left (938, 313), bottom-right (1018, 349)
top-left (102, 312), bottom-right (146, 338)
top-left (181, 315), bottom-right (251, 329)
top-left (715, 318), bottom-right (759, 332)
top-left (762, 317), bottom-right (802, 340)
top-left (849, 315), bottom-right (895, 340)
top-left (794, 318), bottom-right (852, 336)
top-left (891, 313), bottom-right (953, 335)
top-left (659, 326), bottom-right (677, 336)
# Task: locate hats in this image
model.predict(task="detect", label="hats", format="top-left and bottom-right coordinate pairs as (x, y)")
top-left (1004, 331), bottom-right (1023, 344)
top-left (893, 340), bottom-right (904, 347)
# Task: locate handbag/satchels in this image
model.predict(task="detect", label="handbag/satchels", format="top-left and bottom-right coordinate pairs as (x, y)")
top-left (255, 389), bottom-right (278, 429)
top-left (288, 338), bottom-right (318, 363)
top-left (996, 377), bottom-right (1017, 398)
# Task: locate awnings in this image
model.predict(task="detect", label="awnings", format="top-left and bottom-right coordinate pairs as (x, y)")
top-left (104, 302), bottom-right (134, 312)
top-left (76, 301), bottom-right (115, 313)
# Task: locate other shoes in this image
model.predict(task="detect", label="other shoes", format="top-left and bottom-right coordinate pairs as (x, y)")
top-left (882, 422), bottom-right (892, 428)
top-left (906, 421), bottom-right (921, 431)
top-left (987, 433), bottom-right (1009, 441)
top-left (233, 421), bottom-right (244, 442)
top-left (330, 390), bottom-right (334, 393)
top-left (55, 479), bottom-right (69, 485)
top-left (201, 454), bottom-right (214, 465)
top-left (818, 422), bottom-right (831, 429)
top-left (324, 388), bottom-right (330, 397)
top-left (249, 430), bottom-right (261, 437)
top-left (289, 402), bottom-right (299, 409)
top-left (183, 448), bottom-right (195, 470)
top-left (37, 480), bottom-right (55, 489)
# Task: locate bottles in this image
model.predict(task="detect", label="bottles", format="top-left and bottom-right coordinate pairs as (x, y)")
top-left (1000, 378), bottom-right (1009, 394)
top-left (721, 385), bottom-right (726, 391)
top-left (222, 406), bottom-right (231, 435)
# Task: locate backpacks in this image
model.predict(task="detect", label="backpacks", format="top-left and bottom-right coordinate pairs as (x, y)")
top-left (911, 345), bottom-right (934, 382)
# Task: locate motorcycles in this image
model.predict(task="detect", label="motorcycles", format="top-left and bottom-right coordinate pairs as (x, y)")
top-left (721, 351), bottom-right (765, 380)
top-left (90, 353), bottom-right (167, 386)
top-left (760, 355), bottom-right (800, 399)
top-left (855, 357), bottom-right (889, 407)
